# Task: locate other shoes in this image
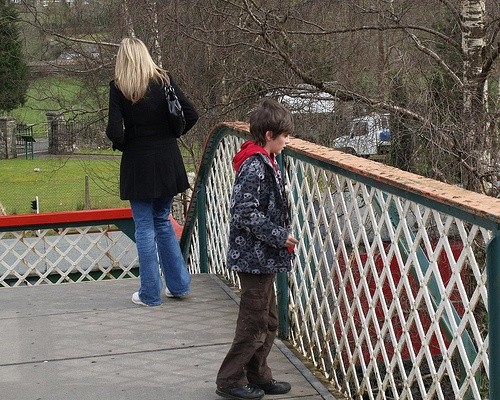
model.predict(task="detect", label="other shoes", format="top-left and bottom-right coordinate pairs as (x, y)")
top-left (260, 379), bottom-right (291, 394)
top-left (216, 384), bottom-right (264, 400)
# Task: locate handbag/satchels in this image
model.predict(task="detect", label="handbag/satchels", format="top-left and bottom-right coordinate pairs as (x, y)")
top-left (164, 83), bottom-right (186, 138)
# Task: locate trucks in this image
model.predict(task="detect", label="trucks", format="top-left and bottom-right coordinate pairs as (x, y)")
top-left (332, 114), bottom-right (391, 157)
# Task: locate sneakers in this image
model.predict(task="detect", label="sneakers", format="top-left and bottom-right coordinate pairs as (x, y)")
top-left (165, 287), bottom-right (174, 297)
top-left (132, 292), bottom-right (149, 307)
